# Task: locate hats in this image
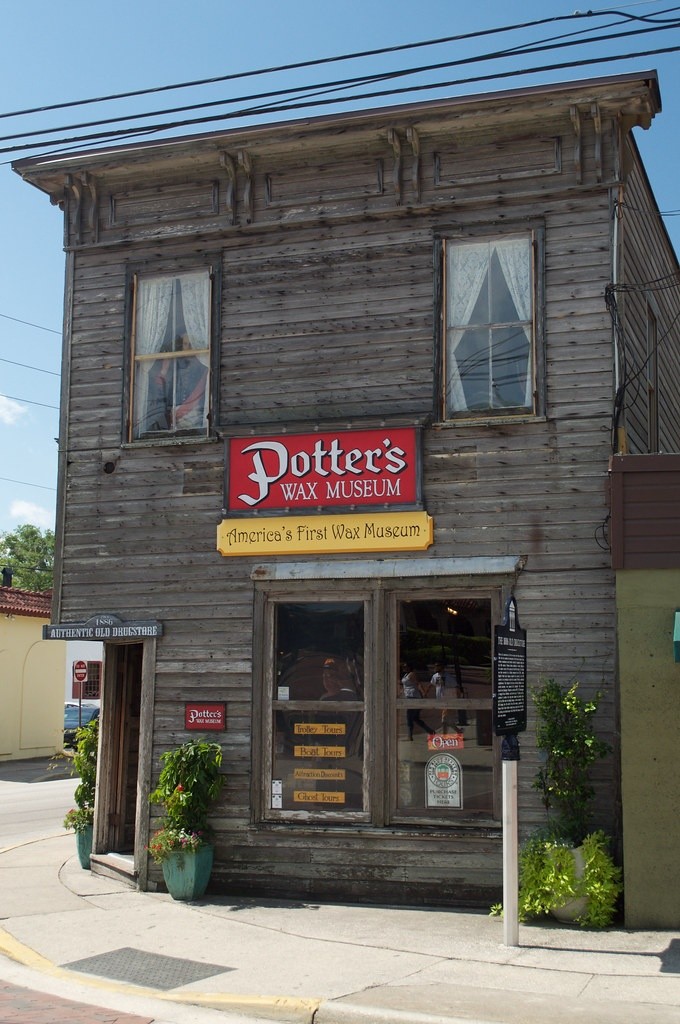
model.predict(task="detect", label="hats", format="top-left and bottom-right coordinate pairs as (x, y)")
top-left (278, 648), bottom-right (291, 659)
top-left (320, 658), bottom-right (351, 674)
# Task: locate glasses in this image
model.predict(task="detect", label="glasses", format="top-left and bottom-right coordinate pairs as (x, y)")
top-left (400, 665), bottom-right (406, 669)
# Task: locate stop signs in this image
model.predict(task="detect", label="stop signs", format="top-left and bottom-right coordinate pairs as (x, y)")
top-left (74, 660), bottom-right (87, 681)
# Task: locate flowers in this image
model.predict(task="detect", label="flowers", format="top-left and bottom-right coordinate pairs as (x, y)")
top-left (64, 808), bottom-right (93, 832)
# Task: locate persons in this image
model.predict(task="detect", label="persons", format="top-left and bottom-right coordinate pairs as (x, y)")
top-left (160, 334), bottom-right (204, 428)
top-left (397, 659), bottom-right (464, 740)
top-left (315, 658), bottom-right (359, 744)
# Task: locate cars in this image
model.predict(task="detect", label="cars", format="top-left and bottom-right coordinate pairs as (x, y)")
top-left (63, 701), bottom-right (99, 751)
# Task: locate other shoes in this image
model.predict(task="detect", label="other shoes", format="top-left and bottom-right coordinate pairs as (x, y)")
top-left (425, 730), bottom-right (436, 742)
top-left (403, 737), bottom-right (413, 741)
top-left (456, 728), bottom-right (464, 734)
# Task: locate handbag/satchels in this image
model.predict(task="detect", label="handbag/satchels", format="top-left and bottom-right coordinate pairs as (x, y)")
top-left (416, 681), bottom-right (427, 699)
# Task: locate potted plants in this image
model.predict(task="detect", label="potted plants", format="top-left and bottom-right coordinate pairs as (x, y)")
top-left (143, 785), bottom-right (207, 864)
top-left (488, 678), bottom-right (624, 927)
top-left (47, 714), bottom-right (98, 870)
top-left (147, 737), bottom-right (227, 900)
